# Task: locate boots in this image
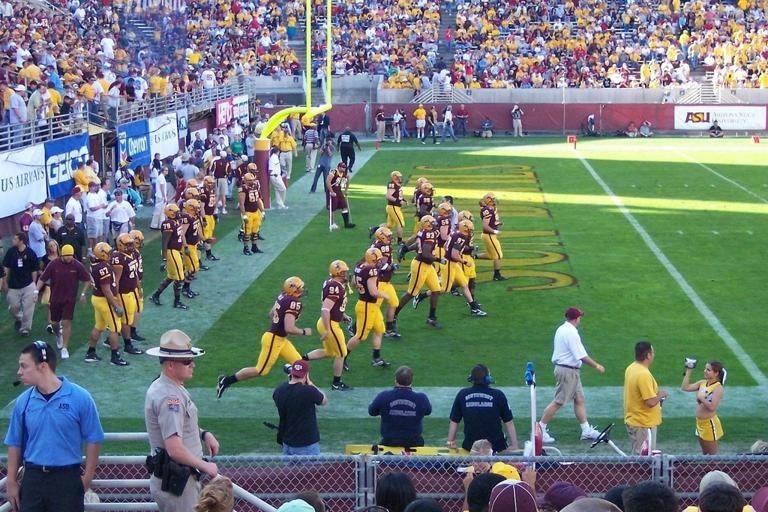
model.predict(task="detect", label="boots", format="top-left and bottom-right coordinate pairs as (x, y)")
top-left (341, 212), bottom-right (356, 228)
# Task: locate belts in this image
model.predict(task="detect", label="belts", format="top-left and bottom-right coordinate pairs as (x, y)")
top-left (269, 173), bottom-right (279, 177)
top-left (21, 459), bottom-right (81, 473)
top-left (281, 150), bottom-right (292, 153)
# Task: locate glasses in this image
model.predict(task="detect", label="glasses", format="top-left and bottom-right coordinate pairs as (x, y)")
top-left (166, 357), bottom-right (194, 365)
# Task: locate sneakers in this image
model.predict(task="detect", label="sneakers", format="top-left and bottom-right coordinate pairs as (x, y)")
top-left (14, 319), bottom-right (21, 331)
top-left (214, 213), bottom-right (220, 224)
top-left (101, 340), bottom-right (110, 349)
top-left (202, 218), bottom-right (208, 227)
top-left (396, 247), bottom-right (404, 263)
top-left (109, 357), bottom-right (129, 367)
top-left (221, 207), bottom-right (228, 215)
top-left (243, 248), bottom-right (252, 256)
top-left (343, 362), bottom-right (349, 371)
top-left (384, 329), bottom-right (402, 338)
top-left (123, 346), bottom-right (143, 355)
top-left (579, 428), bottom-right (607, 442)
top-left (470, 307), bottom-right (487, 318)
top-left (392, 314), bottom-right (398, 328)
top-left (492, 274), bottom-right (509, 281)
top-left (173, 301), bottom-right (188, 310)
top-left (54, 334), bottom-right (63, 350)
top-left (541, 430), bottom-right (556, 444)
top-left (21, 328), bottom-right (30, 337)
top-left (427, 318), bottom-right (441, 329)
top-left (258, 233), bottom-right (265, 242)
top-left (238, 232), bottom-right (244, 241)
top-left (451, 290), bottom-right (464, 298)
top-left (182, 288), bottom-right (194, 298)
top-left (206, 254), bottom-right (216, 261)
top-left (251, 246), bottom-right (264, 254)
top-left (332, 382), bottom-right (354, 392)
top-left (148, 295), bottom-right (162, 306)
top-left (200, 266), bottom-right (211, 271)
top-left (188, 288), bottom-right (200, 296)
top-left (129, 334), bottom-right (145, 343)
top-left (371, 358), bottom-right (392, 369)
top-left (83, 354), bottom-right (102, 364)
top-left (345, 325), bottom-right (355, 335)
top-left (412, 293), bottom-right (421, 310)
top-left (45, 323), bottom-right (52, 333)
top-left (214, 375), bottom-right (230, 398)
top-left (214, 208), bottom-right (219, 215)
top-left (60, 347), bottom-right (69, 360)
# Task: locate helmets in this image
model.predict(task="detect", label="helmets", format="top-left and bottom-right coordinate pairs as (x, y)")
top-left (282, 275), bottom-right (305, 299)
top-left (327, 258), bottom-right (350, 281)
top-left (247, 163), bottom-right (258, 174)
top-left (203, 175), bottom-right (215, 189)
top-left (243, 173), bottom-right (256, 186)
top-left (459, 220), bottom-right (474, 237)
top-left (94, 241), bottom-right (112, 262)
top-left (45, 197), bottom-right (54, 205)
top-left (458, 209), bottom-right (473, 221)
top-left (185, 198), bottom-right (200, 216)
top-left (373, 227), bottom-right (393, 244)
top-left (129, 228), bottom-right (146, 249)
top-left (420, 183), bottom-right (435, 196)
top-left (391, 171), bottom-right (403, 184)
top-left (364, 247), bottom-right (383, 267)
top-left (116, 232), bottom-right (134, 254)
top-left (484, 194), bottom-right (496, 205)
top-left (420, 215), bottom-right (438, 231)
top-left (416, 178), bottom-right (428, 189)
top-left (164, 203), bottom-right (180, 219)
top-left (185, 187), bottom-right (200, 200)
top-left (187, 179), bottom-right (199, 190)
top-left (438, 202), bottom-right (454, 219)
top-left (119, 178), bottom-right (129, 185)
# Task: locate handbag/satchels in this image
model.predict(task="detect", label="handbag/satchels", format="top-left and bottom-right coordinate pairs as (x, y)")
top-left (112, 221), bottom-right (122, 232)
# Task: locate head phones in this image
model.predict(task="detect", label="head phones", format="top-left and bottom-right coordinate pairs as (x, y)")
top-left (33, 340), bottom-right (48, 361)
top-left (466, 365), bottom-right (494, 386)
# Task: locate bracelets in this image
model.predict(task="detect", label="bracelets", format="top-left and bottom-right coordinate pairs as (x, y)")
top-left (202, 430), bottom-right (210, 440)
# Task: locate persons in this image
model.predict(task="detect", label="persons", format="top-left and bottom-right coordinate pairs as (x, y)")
top-left (216, 276), bottom-right (312, 397)
top-left (368, 365), bottom-right (432, 447)
top-left (586, 113), bottom-right (653, 139)
top-left (4, 341), bottom-right (105, 512)
top-left (303, 259), bottom-right (352, 391)
top-left (539, 307), bottom-right (605, 444)
top-left (273, 359), bottom-right (327, 465)
top-left (447, 365), bottom-right (517, 456)
top-left (623, 342), bottom-right (667, 456)
top-left (682, 361), bottom-right (727, 455)
top-left (145, 328), bottom-right (219, 512)
top-left (192, 439), bottom-right (768, 511)
top-left (2, 1), bottom-right (305, 150)
top-left (374, 104), bottom-right (526, 145)
top-left (311, 0), bottom-right (768, 103)
top-left (343, 170), bottom-right (507, 369)
top-left (1, 110), bottom-right (362, 366)
top-left (710, 120), bottom-right (724, 138)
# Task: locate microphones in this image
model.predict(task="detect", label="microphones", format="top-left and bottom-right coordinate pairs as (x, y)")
top-left (13, 380), bottom-right (23, 387)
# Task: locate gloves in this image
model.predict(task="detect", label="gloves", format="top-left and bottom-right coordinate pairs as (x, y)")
top-left (261, 211), bottom-right (266, 223)
top-left (441, 259), bottom-right (447, 265)
top-left (184, 246), bottom-right (191, 257)
top-left (159, 259), bottom-right (168, 273)
top-left (493, 228), bottom-right (501, 235)
top-left (391, 262), bottom-right (400, 272)
top-left (204, 237), bottom-right (217, 245)
top-left (113, 303), bottom-right (124, 318)
top-left (243, 213), bottom-right (249, 221)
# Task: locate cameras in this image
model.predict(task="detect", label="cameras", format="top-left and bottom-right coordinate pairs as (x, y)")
top-left (282, 362), bottom-right (293, 374)
top-left (325, 143), bottom-right (329, 147)
top-left (683, 358), bottom-right (699, 369)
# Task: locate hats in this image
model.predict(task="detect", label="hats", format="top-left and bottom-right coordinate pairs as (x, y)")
top-left (309, 122), bottom-right (317, 128)
top-left (49, 205), bottom-right (64, 215)
top-left (272, 498), bottom-right (315, 512)
top-left (291, 360), bottom-right (308, 377)
top-left (219, 150), bottom-right (227, 158)
top-left (490, 461), bottom-right (522, 481)
top-left (114, 189), bottom-right (123, 195)
top-left (32, 209), bottom-right (45, 218)
top-left (338, 162), bottom-right (347, 168)
top-left (564, 307), bottom-right (583, 319)
top-left (465, 474), bottom-right (507, 507)
top-left (751, 486), bottom-right (768, 512)
top-left (88, 181), bottom-right (97, 189)
top-left (59, 244), bottom-right (75, 258)
top-left (489, 478), bottom-right (536, 512)
top-left (72, 187), bottom-right (81, 195)
top-left (181, 153), bottom-right (190, 163)
top-left (143, 328), bottom-right (207, 359)
top-left (14, 84), bottom-right (26, 92)
top-left (537, 478), bottom-right (587, 506)
top-left (25, 203), bottom-right (33, 210)
top-left (242, 154), bottom-right (249, 162)
top-left (64, 214), bottom-right (75, 225)
top-left (700, 470), bottom-right (738, 491)
